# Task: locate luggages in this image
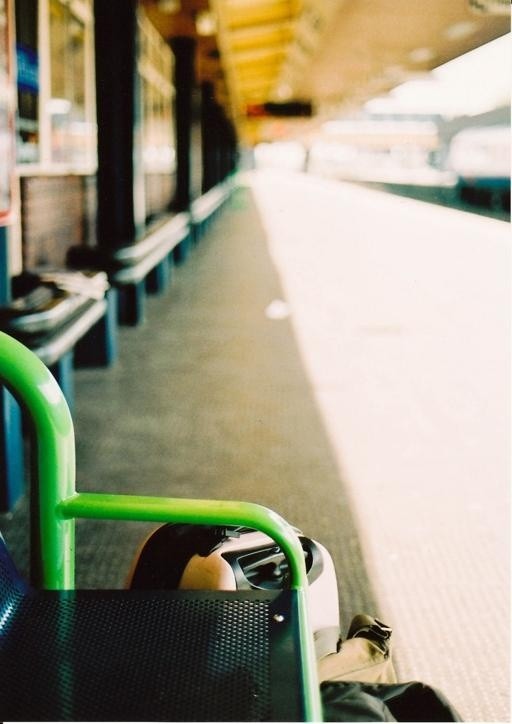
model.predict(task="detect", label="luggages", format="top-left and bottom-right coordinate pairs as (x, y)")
top-left (123, 517), bottom-right (343, 658)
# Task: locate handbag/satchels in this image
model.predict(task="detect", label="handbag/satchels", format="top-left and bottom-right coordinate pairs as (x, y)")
top-left (315, 614), bottom-right (466, 723)
